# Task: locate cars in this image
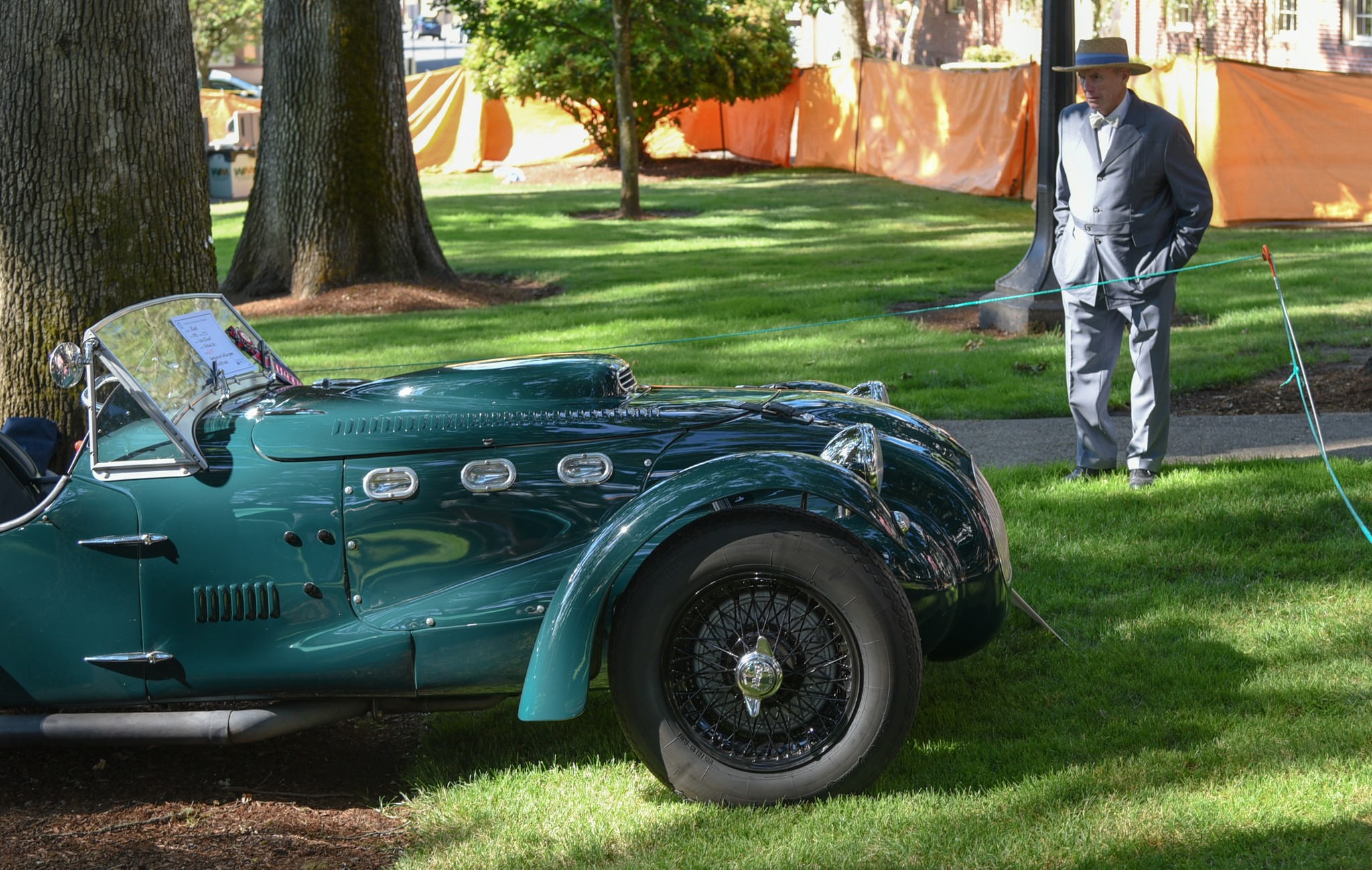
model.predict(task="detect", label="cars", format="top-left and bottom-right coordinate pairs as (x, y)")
top-left (195, 68), bottom-right (262, 99)
top-left (410, 15), bottom-right (441, 42)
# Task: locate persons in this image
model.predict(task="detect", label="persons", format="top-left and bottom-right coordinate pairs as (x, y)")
top-left (1051, 37), bottom-right (1213, 487)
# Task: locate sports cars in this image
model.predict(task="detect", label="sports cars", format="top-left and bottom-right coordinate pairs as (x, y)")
top-left (0, 289), bottom-right (1016, 811)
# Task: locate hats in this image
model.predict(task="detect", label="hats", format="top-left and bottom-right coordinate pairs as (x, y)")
top-left (1052, 37), bottom-right (1152, 75)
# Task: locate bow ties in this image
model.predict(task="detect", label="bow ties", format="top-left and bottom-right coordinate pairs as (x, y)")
top-left (1088, 113), bottom-right (1119, 130)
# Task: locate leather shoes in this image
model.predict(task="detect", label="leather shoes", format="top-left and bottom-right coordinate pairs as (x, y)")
top-left (1063, 465), bottom-right (1115, 483)
top-left (1128, 468), bottom-right (1154, 490)
